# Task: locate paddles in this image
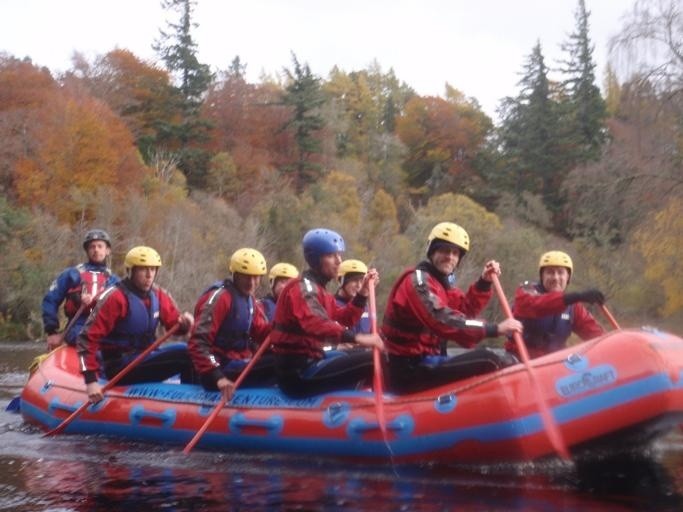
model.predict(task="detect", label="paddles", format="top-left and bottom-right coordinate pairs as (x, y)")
top-left (41, 322), bottom-right (179, 438)
top-left (366, 277), bottom-right (384, 432)
top-left (489, 271), bottom-right (568, 460)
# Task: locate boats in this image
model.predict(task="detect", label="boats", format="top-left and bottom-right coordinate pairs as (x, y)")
top-left (11, 318), bottom-right (683, 472)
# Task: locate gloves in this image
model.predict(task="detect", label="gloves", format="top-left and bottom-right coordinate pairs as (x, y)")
top-left (564, 290), bottom-right (606, 308)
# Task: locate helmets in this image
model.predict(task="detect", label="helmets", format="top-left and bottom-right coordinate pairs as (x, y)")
top-left (302, 228), bottom-right (345, 270)
top-left (537, 250), bottom-right (572, 284)
top-left (268, 262), bottom-right (298, 288)
top-left (124, 245), bottom-right (162, 281)
top-left (82, 230), bottom-right (111, 249)
top-left (335, 259), bottom-right (368, 288)
top-left (228, 248), bottom-right (266, 283)
top-left (426, 222), bottom-right (471, 259)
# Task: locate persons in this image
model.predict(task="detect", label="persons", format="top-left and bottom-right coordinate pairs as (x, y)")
top-left (254, 260), bottom-right (302, 320)
top-left (42, 229), bottom-right (119, 354)
top-left (332, 258), bottom-right (375, 353)
top-left (184, 248), bottom-right (277, 407)
top-left (274, 227), bottom-right (393, 398)
top-left (77, 246), bottom-right (194, 403)
top-left (506, 247), bottom-right (606, 360)
top-left (380, 215), bottom-right (524, 397)
